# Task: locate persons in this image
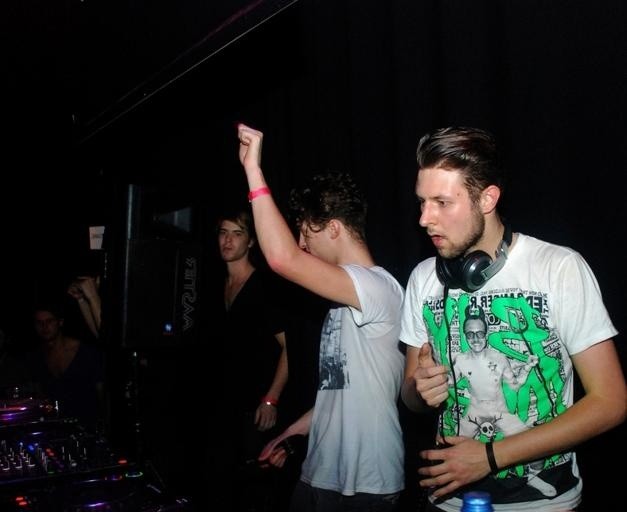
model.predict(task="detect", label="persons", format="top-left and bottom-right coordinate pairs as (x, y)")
top-left (67, 276), bottom-right (101, 336)
top-left (399, 125), bottom-right (626, 511)
top-left (236, 121), bottom-right (406, 511)
top-left (35, 309), bottom-right (70, 370)
top-left (217, 210), bottom-right (289, 434)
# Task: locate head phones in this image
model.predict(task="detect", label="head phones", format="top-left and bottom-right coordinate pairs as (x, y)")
top-left (435, 221), bottom-right (512, 293)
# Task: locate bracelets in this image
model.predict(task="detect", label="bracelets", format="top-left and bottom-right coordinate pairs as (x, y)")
top-left (262, 396), bottom-right (279, 407)
top-left (485, 442), bottom-right (498, 474)
top-left (247, 186), bottom-right (273, 202)
